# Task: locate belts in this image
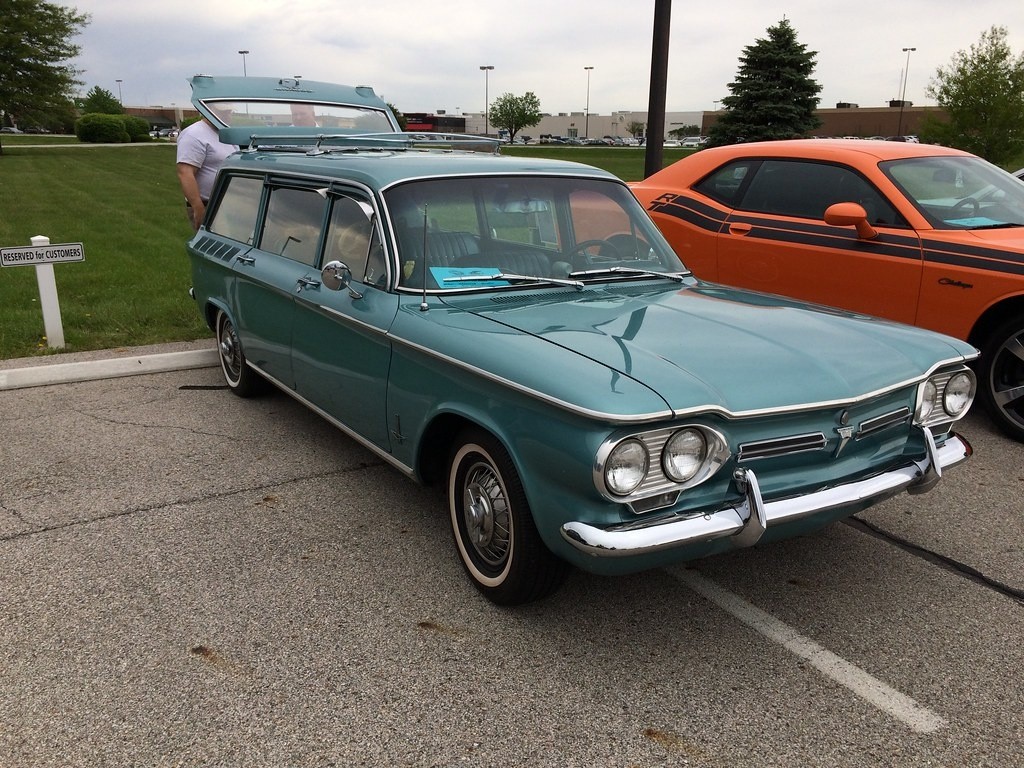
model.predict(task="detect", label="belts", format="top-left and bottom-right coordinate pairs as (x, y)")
top-left (186, 200), bottom-right (208, 207)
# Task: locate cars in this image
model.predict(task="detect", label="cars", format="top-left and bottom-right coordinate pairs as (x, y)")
top-left (811, 135), bottom-right (920, 145)
top-left (175, 74), bottom-right (981, 608)
top-left (23, 125), bottom-right (51, 134)
top-left (554, 137), bottom-right (1024, 446)
top-left (149, 128), bottom-right (181, 138)
top-left (541, 135), bottom-right (711, 148)
top-left (0, 126), bottom-right (24, 134)
top-left (916, 168), bottom-right (1024, 224)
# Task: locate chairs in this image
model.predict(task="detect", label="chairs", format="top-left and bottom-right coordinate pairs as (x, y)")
top-left (452, 248), bottom-right (551, 279)
top-left (840, 171), bottom-right (879, 224)
top-left (747, 170), bottom-right (783, 211)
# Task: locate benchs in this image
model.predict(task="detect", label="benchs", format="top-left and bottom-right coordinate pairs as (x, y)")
top-left (416, 230), bottom-right (479, 288)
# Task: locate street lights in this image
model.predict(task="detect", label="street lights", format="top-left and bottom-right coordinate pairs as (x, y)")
top-left (584, 66), bottom-right (594, 138)
top-left (896, 48), bottom-right (917, 138)
top-left (115, 79), bottom-right (123, 106)
top-left (238, 50), bottom-right (250, 76)
top-left (479, 66), bottom-right (495, 138)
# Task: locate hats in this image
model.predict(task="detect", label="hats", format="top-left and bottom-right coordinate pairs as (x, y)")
top-left (211, 102), bottom-right (234, 111)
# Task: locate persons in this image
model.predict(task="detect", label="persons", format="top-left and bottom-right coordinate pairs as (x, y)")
top-left (177, 96), bottom-right (242, 230)
top-left (268, 102), bottom-right (328, 224)
top-left (157, 131), bottom-right (159, 139)
